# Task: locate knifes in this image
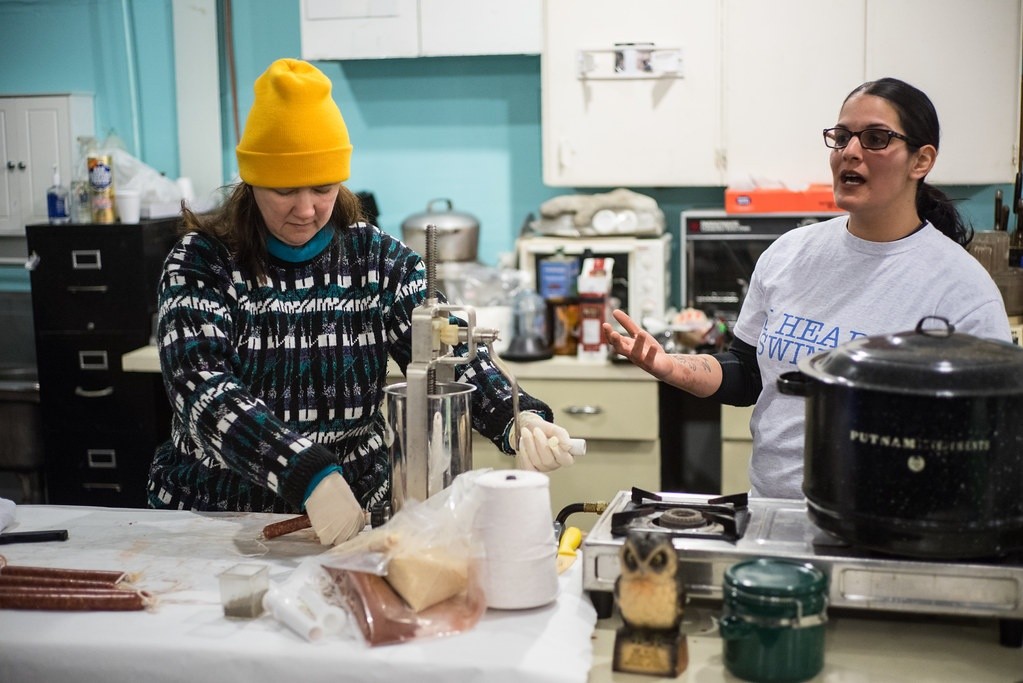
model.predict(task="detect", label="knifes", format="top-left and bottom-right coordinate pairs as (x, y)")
top-left (993, 172), bottom-right (1023, 247)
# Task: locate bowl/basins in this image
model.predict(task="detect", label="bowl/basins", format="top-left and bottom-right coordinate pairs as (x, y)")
top-left (500, 333), bottom-right (554, 363)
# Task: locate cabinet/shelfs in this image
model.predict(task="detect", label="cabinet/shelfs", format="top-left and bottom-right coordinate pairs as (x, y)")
top-left (123, 330), bottom-right (664, 531)
top-left (0, 94), bottom-right (97, 237)
top-left (296, 2), bottom-right (1019, 190)
top-left (25, 212), bottom-right (186, 511)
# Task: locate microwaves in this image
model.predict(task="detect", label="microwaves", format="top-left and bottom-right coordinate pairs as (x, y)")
top-left (516, 232), bottom-right (672, 337)
top-left (680, 208), bottom-right (850, 338)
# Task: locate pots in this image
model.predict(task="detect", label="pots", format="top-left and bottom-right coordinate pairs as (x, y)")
top-left (401, 198), bottom-right (481, 263)
top-left (775, 314), bottom-right (1023, 558)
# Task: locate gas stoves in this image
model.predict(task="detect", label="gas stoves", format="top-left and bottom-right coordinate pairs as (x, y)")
top-left (580, 486), bottom-right (1023, 648)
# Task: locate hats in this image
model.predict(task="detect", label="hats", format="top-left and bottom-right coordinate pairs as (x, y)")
top-left (235, 57), bottom-right (352, 190)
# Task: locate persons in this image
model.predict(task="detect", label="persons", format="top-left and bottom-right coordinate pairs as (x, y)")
top-left (145, 58), bottom-right (575, 547)
top-left (601, 78), bottom-right (1012, 500)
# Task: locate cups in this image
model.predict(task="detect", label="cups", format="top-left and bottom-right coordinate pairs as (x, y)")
top-left (383, 381), bottom-right (477, 517)
top-left (115, 189), bottom-right (142, 225)
top-left (720, 557), bottom-right (828, 683)
top-left (965, 231), bottom-right (1023, 316)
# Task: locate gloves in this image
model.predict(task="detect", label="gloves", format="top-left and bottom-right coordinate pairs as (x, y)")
top-left (509, 410), bottom-right (576, 472)
top-left (303, 471), bottom-right (366, 546)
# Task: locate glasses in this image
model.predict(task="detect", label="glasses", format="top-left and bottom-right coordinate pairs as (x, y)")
top-left (823, 128), bottom-right (922, 150)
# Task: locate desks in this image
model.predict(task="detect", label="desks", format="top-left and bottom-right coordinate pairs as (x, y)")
top-left (0, 494), bottom-right (1023, 682)
